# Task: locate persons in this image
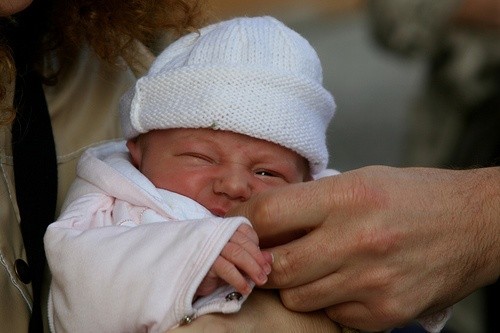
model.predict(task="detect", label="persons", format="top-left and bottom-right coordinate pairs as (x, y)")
top-left (44, 16), bottom-right (350, 333)
top-left (0, 0), bottom-right (500, 333)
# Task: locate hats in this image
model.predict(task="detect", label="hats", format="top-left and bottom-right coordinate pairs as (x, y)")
top-left (118, 16), bottom-right (336, 176)
